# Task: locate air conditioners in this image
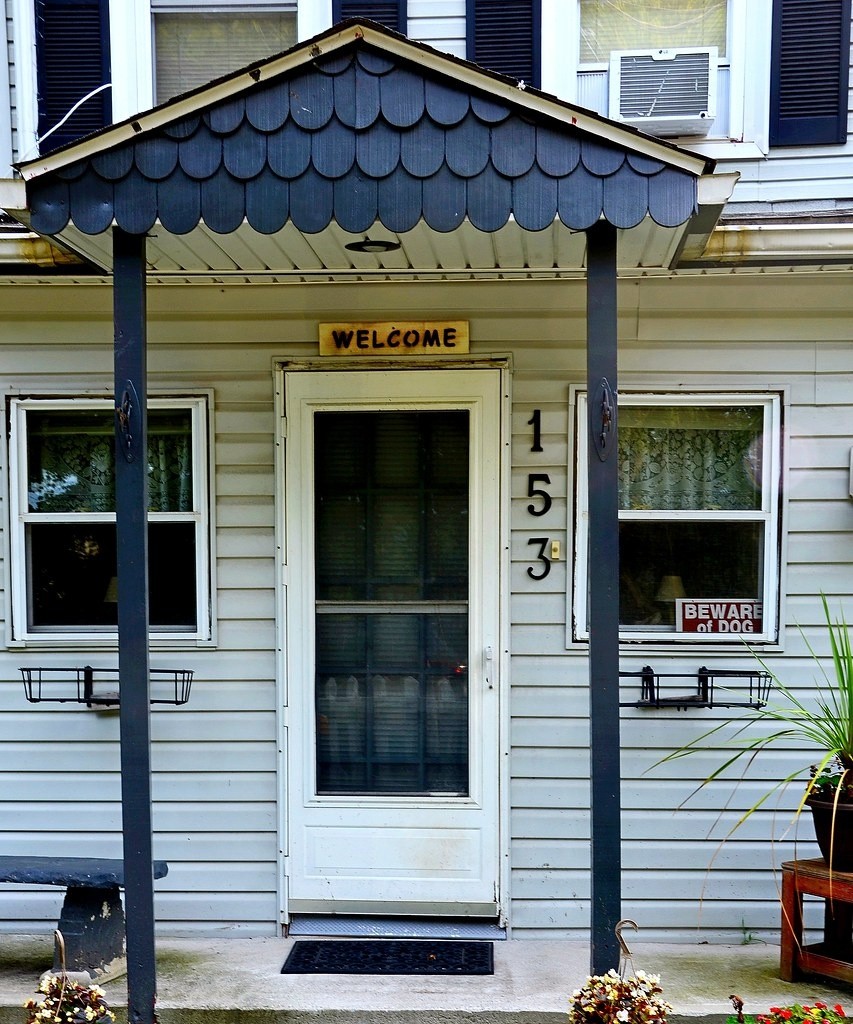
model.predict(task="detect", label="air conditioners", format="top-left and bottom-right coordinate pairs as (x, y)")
top-left (608, 44), bottom-right (720, 136)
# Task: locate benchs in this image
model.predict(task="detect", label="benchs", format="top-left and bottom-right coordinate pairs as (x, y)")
top-left (0, 856), bottom-right (170, 995)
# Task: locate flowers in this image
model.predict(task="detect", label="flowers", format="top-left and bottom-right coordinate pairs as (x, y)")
top-left (756, 1003), bottom-right (846, 1024)
top-left (22, 973), bottom-right (116, 1023)
top-left (567, 966), bottom-right (678, 1024)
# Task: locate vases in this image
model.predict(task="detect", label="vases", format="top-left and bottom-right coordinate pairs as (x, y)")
top-left (29, 998), bottom-right (116, 1023)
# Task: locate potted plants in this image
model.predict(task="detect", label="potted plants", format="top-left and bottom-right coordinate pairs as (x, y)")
top-left (641, 592), bottom-right (853, 874)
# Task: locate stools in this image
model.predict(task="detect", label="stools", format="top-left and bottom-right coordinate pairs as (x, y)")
top-left (779, 858), bottom-right (853, 983)
